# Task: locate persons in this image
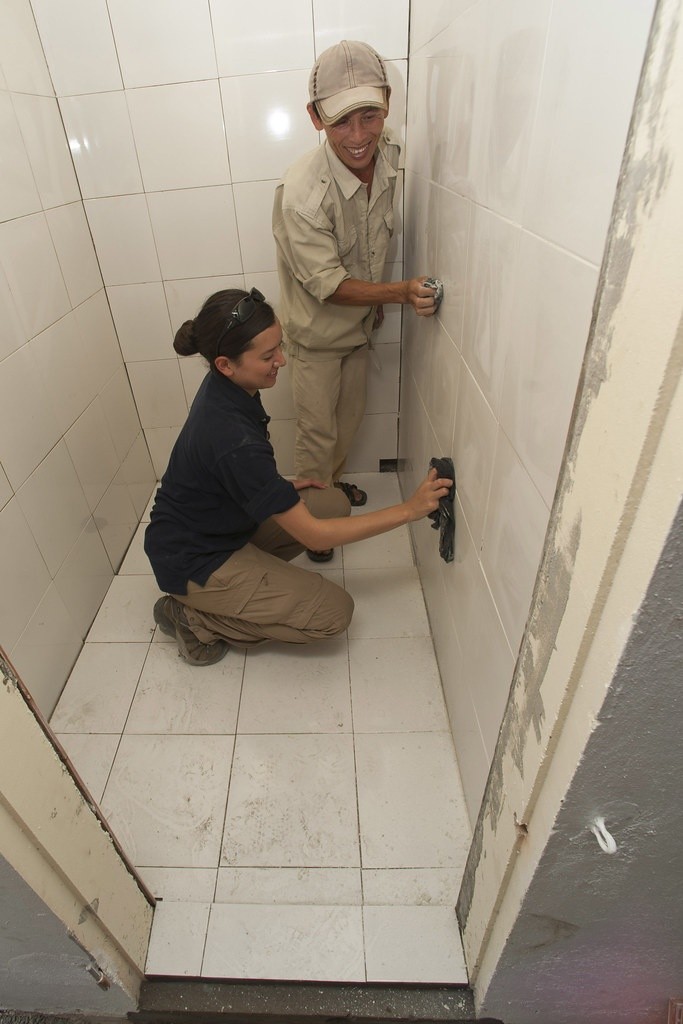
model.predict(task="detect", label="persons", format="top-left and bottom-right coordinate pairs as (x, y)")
top-left (144, 287), bottom-right (452, 667)
top-left (271, 39), bottom-right (439, 565)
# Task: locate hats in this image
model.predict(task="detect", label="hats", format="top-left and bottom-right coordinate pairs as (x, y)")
top-left (308, 40), bottom-right (390, 125)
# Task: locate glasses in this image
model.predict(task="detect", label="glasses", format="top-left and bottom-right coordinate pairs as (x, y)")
top-left (216, 287), bottom-right (265, 358)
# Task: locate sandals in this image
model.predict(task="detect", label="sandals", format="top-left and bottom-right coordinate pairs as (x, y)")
top-left (340, 482), bottom-right (367, 506)
top-left (306, 548), bottom-right (334, 561)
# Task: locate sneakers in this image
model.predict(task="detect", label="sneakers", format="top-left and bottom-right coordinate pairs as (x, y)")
top-left (154, 595), bottom-right (229, 665)
